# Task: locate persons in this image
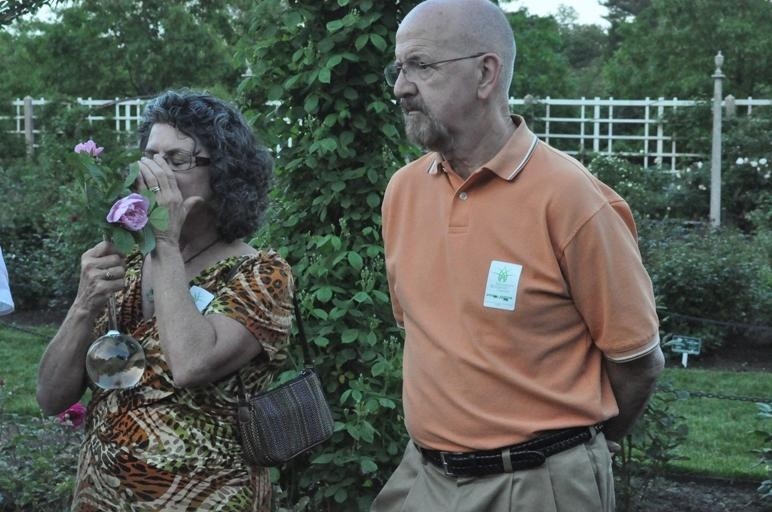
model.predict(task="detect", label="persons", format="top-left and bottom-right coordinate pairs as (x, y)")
top-left (365, 0), bottom-right (666, 512)
top-left (36, 89), bottom-right (298, 512)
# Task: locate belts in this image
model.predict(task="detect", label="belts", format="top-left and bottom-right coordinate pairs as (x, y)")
top-left (413, 424), bottom-right (605, 478)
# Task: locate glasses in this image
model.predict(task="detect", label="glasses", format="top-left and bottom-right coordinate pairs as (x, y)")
top-left (383, 53), bottom-right (481, 88)
top-left (143, 150), bottom-right (209, 172)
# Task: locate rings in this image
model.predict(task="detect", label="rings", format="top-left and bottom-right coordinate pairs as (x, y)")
top-left (105, 269), bottom-right (113, 279)
top-left (148, 185), bottom-right (161, 192)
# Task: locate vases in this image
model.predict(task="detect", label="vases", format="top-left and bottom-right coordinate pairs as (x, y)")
top-left (85, 233), bottom-right (147, 390)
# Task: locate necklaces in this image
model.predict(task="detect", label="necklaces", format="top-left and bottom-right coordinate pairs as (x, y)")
top-left (143, 237), bottom-right (230, 303)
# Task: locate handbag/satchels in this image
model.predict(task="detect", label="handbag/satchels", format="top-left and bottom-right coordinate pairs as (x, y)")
top-left (236, 370), bottom-right (335, 467)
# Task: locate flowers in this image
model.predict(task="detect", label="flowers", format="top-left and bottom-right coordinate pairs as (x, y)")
top-left (55, 141), bottom-right (172, 256)
